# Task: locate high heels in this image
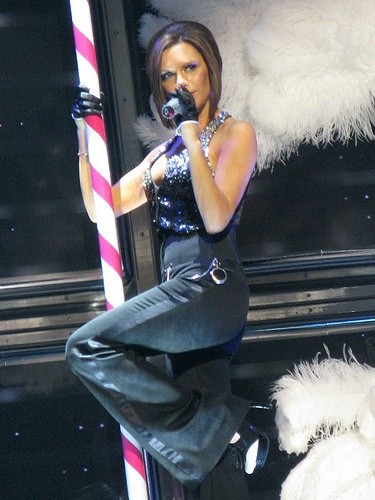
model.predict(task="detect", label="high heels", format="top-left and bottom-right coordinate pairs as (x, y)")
top-left (225, 399), bottom-right (272, 475)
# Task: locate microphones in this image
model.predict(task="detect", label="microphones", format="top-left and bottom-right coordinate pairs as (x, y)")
top-left (161, 98), bottom-right (182, 119)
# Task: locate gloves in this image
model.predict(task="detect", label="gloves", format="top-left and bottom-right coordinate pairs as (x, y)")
top-left (170, 86), bottom-right (200, 136)
top-left (72, 85), bottom-right (105, 120)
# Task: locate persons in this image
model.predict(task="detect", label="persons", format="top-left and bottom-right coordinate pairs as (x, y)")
top-left (71, 21), bottom-right (272, 500)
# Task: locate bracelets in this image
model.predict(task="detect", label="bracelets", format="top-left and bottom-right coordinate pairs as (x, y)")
top-left (77, 152), bottom-right (88, 156)
